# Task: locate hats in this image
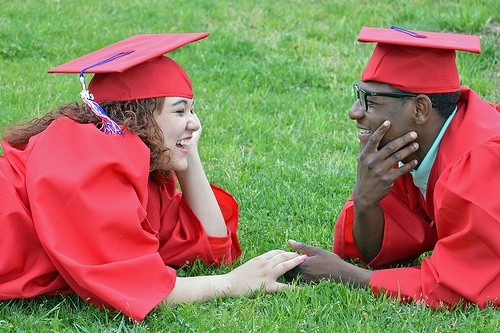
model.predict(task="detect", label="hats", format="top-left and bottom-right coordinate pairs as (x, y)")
top-left (356, 26), bottom-right (480, 93)
top-left (47, 32), bottom-right (209, 135)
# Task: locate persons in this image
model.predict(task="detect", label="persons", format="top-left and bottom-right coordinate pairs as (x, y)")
top-left (282, 24), bottom-right (500, 313)
top-left (0, 32), bottom-right (308, 323)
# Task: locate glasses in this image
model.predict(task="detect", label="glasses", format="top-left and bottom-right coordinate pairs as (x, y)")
top-left (353, 83), bottom-right (437, 112)
